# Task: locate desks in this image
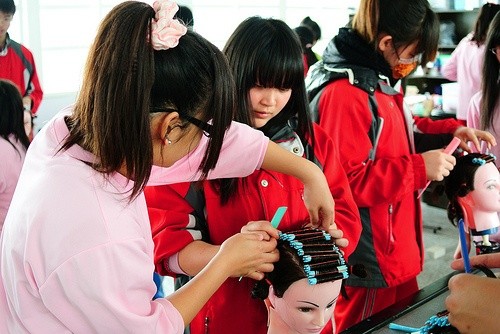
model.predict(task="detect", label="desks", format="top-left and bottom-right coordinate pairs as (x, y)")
top-left (339, 266), bottom-right (500, 334)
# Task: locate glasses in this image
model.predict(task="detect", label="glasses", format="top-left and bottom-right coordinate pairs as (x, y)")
top-left (488, 46), bottom-right (500, 55)
top-left (151, 107), bottom-right (218, 137)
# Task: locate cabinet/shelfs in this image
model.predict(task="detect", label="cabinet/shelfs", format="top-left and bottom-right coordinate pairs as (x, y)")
top-left (400, 11), bottom-right (466, 95)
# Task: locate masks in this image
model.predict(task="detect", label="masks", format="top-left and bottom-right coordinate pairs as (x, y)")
top-left (388, 43), bottom-right (417, 79)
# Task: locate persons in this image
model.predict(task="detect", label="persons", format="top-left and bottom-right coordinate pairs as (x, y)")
top-left (0, 0), bottom-right (362, 334)
top-left (251, 228), bottom-right (350, 334)
top-left (440, 2), bottom-right (500, 334)
top-left (443, 153), bottom-right (500, 236)
top-left (306, 1), bottom-right (497, 334)
